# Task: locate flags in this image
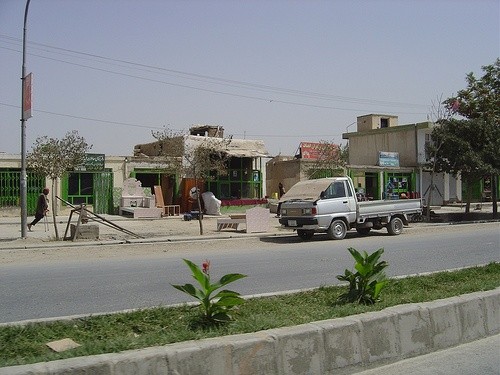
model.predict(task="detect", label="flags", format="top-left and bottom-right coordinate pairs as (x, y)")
top-left (23, 74), bottom-right (32, 121)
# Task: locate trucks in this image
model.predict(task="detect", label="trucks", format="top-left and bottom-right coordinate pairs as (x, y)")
top-left (276, 175), bottom-right (422, 241)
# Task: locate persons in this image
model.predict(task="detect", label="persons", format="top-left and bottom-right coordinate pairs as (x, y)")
top-left (27, 189), bottom-right (50, 231)
top-left (275, 182), bottom-right (286, 218)
